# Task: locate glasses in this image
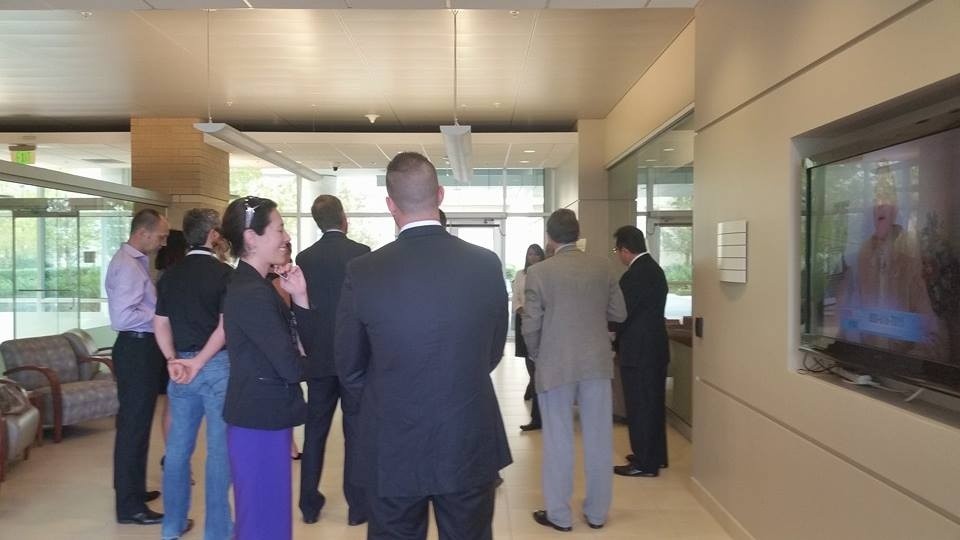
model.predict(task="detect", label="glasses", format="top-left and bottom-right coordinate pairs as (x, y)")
top-left (243, 195), bottom-right (260, 229)
top-left (613, 248), bottom-right (621, 254)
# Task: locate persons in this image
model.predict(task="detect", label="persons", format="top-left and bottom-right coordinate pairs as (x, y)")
top-left (266, 241), bottom-right (304, 460)
top-left (519, 241), bottom-right (555, 432)
top-left (295, 194), bottom-right (371, 527)
top-left (519, 208), bottom-right (628, 533)
top-left (511, 244), bottom-right (547, 402)
top-left (332, 151), bottom-right (515, 540)
top-left (103, 209), bottom-right (171, 526)
top-left (154, 207), bottom-right (236, 540)
top-left (822, 155), bottom-right (943, 363)
top-left (606, 225), bottom-right (670, 477)
top-left (221, 195), bottom-right (315, 540)
top-left (154, 228), bottom-right (195, 487)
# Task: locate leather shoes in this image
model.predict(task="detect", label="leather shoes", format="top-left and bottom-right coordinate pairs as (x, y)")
top-left (626, 454), bottom-right (668, 468)
top-left (614, 464), bottom-right (658, 477)
top-left (118, 509), bottom-right (164, 525)
top-left (304, 490), bottom-right (325, 523)
top-left (144, 490), bottom-right (160, 503)
top-left (533, 510), bottom-right (572, 531)
top-left (584, 513), bottom-right (604, 528)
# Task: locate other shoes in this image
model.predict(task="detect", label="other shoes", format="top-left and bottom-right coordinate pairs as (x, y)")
top-left (291, 453), bottom-right (303, 460)
top-left (521, 420), bottom-right (541, 431)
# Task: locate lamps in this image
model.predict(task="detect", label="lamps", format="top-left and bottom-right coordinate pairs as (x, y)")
top-left (192, 8), bottom-right (323, 181)
top-left (438, 11), bottom-right (479, 182)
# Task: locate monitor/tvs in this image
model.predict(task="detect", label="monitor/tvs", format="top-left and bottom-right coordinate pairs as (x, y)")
top-left (799, 109), bottom-right (960, 396)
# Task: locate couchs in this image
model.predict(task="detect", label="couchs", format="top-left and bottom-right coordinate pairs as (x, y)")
top-left (1, 335), bottom-right (117, 443)
top-left (0, 376), bottom-right (42, 484)
top-left (62, 327), bottom-right (119, 383)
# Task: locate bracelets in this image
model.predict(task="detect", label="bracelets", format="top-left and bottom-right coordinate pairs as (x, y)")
top-left (166, 354), bottom-right (178, 362)
